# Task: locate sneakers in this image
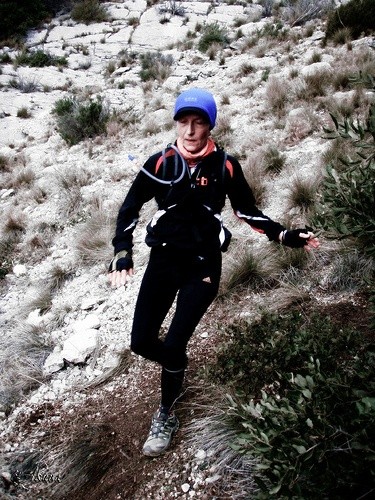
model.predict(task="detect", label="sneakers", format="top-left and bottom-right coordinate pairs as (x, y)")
top-left (181, 352), bottom-right (191, 387)
top-left (141, 408), bottom-right (180, 457)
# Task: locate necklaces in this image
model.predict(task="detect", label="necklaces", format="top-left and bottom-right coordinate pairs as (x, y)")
top-left (185, 161), bottom-right (202, 188)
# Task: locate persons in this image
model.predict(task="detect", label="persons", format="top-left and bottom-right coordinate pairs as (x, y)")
top-left (108, 87), bottom-right (322, 457)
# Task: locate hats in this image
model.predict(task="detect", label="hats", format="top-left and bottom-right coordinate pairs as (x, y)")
top-left (173, 87), bottom-right (217, 128)
